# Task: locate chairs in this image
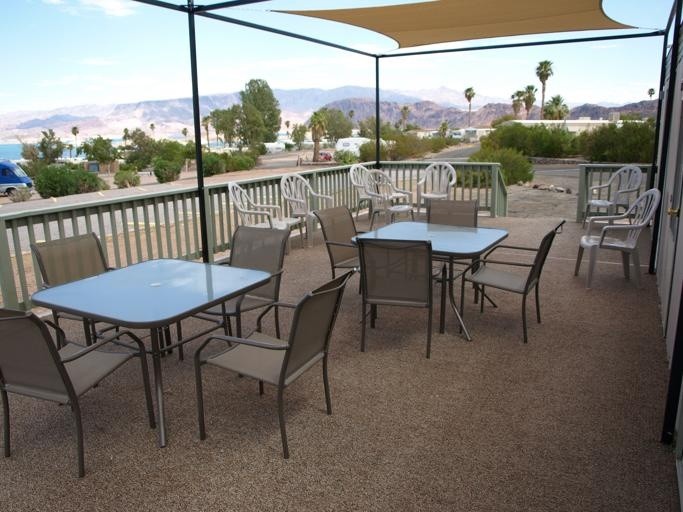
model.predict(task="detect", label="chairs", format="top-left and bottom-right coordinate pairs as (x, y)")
top-left (280, 173), bottom-right (333, 239)
top-left (30, 232), bottom-right (175, 358)
top-left (460, 220), bottom-right (566, 344)
top-left (227, 182), bottom-right (303, 255)
top-left (313, 205), bottom-right (372, 329)
top-left (416, 162), bottom-right (456, 215)
top-left (355, 236), bottom-right (446, 360)
top-left (572, 189), bottom-right (660, 290)
top-left (426, 200), bottom-right (478, 290)
top-left (0, 307), bottom-right (156, 478)
top-left (581, 167), bottom-right (642, 235)
top-left (364, 169), bottom-right (414, 224)
top-left (349, 165), bottom-right (391, 222)
top-left (177, 224), bottom-right (291, 362)
top-left (192, 269), bottom-right (360, 460)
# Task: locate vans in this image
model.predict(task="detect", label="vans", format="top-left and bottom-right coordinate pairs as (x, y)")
top-left (334, 137), bottom-right (373, 158)
top-left (0, 159), bottom-right (33, 197)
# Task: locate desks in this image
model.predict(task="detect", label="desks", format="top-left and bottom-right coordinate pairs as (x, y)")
top-left (30, 258), bottom-right (272, 448)
top-left (351, 221), bottom-right (509, 334)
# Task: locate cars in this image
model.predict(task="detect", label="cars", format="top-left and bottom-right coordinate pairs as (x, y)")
top-left (317, 152), bottom-right (333, 161)
